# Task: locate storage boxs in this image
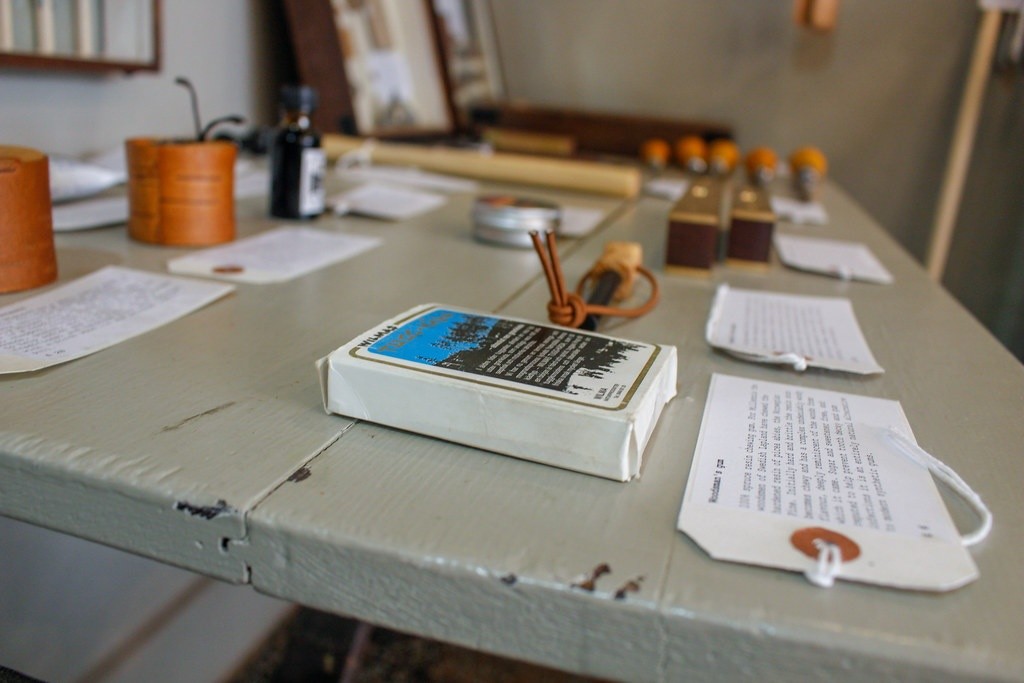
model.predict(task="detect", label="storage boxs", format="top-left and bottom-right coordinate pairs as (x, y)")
top-left (315, 302), bottom-right (678, 483)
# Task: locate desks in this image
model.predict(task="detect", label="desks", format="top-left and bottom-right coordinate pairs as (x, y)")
top-left (0, 131), bottom-right (1024, 683)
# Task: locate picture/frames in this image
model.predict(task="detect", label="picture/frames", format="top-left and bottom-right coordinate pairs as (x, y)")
top-left (431, 0), bottom-right (509, 114)
top-left (285, 0), bottom-right (461, 146)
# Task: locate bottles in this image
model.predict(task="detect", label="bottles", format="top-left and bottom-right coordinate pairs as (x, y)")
top-left (269, 89), bottom-right (325, 220)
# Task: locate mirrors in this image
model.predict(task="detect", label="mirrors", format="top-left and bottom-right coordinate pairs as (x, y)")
top-left (0, 0), bottom-right (161, 78)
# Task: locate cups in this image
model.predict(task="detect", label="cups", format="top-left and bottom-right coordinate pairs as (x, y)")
top-left (125, 137), bottom-right (238, 247)
top-left (0, 145), bottom-right (58, 294)
